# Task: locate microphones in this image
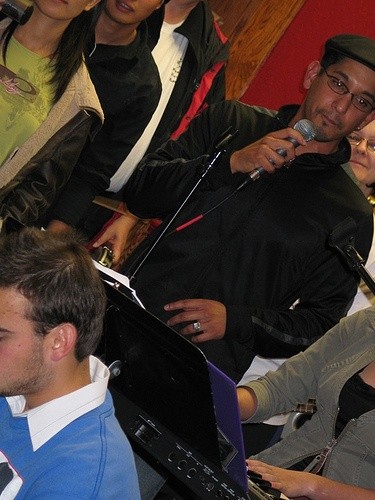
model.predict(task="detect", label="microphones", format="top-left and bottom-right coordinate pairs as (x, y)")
top-left (233, 119), bottom-right (318, 192)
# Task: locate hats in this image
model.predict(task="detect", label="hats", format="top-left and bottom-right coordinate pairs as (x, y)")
top-left (326, 34), bottom-right (375, 70)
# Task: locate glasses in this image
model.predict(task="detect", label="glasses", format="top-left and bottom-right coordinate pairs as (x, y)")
top-left (321, 68), bottom-right (375, 113)
top-left (346, 131), bottom-right (375, 152)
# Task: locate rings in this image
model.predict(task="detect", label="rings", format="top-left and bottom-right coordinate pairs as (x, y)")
top-left (193, 322), bottom-right (200, 334)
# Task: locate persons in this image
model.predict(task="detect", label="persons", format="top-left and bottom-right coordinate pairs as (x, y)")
top-left (124, 32), bottom-right (375, 500)
top-left (0, 229), bottom-right (140, 499)
top-left (0, 0), bottom-right (226, 261)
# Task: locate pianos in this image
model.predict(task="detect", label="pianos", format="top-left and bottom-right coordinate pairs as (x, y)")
top-left (92, 279), bottom-right (291, 500)
